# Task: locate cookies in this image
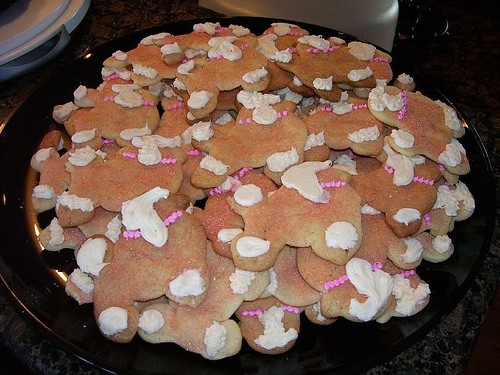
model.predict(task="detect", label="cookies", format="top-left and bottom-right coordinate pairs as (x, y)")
top-left (29, 21), bottom-right (476, 361)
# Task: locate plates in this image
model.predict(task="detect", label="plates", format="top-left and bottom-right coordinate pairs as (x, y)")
top-left (0, 0), bottom-right (90, 80)
top-left (0, 17), bottom-right (499, 374)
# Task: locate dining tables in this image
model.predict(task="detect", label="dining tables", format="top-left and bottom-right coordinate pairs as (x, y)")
top-left (0, 0), bottom-right (499, 374)
